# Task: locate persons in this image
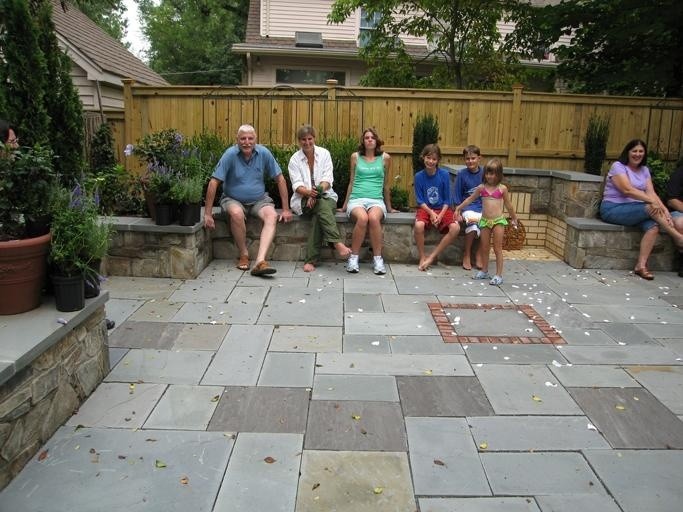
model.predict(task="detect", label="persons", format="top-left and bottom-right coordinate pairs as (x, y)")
top-left (413, 144), bottom-right (460, 270)
top-left (667, 158), bottom-right (682, 277)
top-left (452, 145), bottom-right (485, 269)
top-left (599, 139), bottom-right (682, 280)
top-left (0, 120), bottom-right (20, 158)
top-left (452, 158), bottom-right (518, 285)
top-left (335, 128), bottom-right (400, 274)
top-left (203, 125), bottom-right (293, 276)
top-left (287, 125), bottom-right (352, 271)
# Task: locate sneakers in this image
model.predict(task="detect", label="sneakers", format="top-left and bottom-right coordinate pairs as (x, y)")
top-left (344, 255), bottom-right (360, 273)
top-left (373, 255), bottom-right (386, 275)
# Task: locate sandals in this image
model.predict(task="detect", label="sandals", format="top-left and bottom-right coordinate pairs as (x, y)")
top-left (490, 275), bottom-right (503, 285)
top-left (473, 271), bottom-right (489, 279)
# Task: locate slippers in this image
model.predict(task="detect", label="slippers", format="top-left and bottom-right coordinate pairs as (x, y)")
top-left (251, 260), bottom-right (277, 275)
top-left (238, 255), bottom-right (250, 270)
top-left (635, 268), bottom-right (654, 280)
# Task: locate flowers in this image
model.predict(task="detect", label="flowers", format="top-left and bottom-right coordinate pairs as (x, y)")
top-left (139, 163), bottom-right (174, 195)
top-left (84, 191), bottom-right (114, 261)
top-left (41, 185), bottom-right (107, 292)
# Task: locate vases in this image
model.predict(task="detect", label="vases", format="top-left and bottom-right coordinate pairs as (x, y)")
top-left (141, 192), bottom-right (168, 220)
top-left (85, 257), bottom-right (102, 300)
top-left (154, 200), bottom-right (176, 225)
top-left (47, 265), bottom-right (88, 316)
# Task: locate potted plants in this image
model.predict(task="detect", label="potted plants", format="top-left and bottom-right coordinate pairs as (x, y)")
top-left (0, 132), bottom-right (66, 318)
top-left (176, 181), bottom-right (202, 227)
top-left (193, 182), bottom-right (202, 222)
